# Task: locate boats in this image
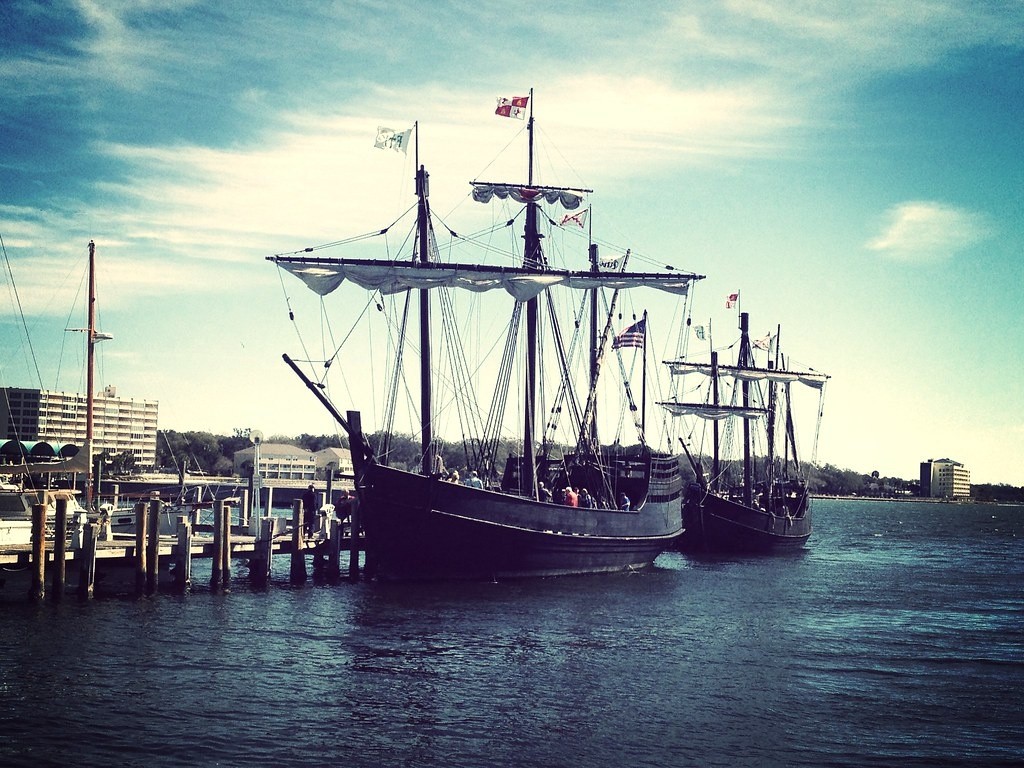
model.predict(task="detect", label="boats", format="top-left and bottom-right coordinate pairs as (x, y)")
top-left (85, 481), bottom-right (200, 536)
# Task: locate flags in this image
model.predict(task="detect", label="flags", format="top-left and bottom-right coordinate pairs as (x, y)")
top-left (610, 319), bottom-right (644, 348)
top-left (374, 126), bottom-right (413, 158)
top-left (692, 325), bottom-right (709, 341)
top-left (558, 208), bottom-right (588, 229)
top-left (724, 293), bottom-right (739, 309)
top-left (751, 334), bottom-right (771, 350)
top-left (494, 95), bottom-right (529, 120)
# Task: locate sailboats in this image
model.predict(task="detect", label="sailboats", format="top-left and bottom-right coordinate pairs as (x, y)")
top-left (1, 238), bottom-right (204, 553)
top-left (263, 83), bottom-right (708, 588)
top-left (657, 305), bottom-right (833, 553)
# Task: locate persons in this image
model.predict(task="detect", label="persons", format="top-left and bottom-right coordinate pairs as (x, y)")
top-left (335, 489), bottom-right (356, 536)
top-left (445, 470), bottom-right (459, 484)
top-left (302, 485), bottom-right (317, 539)
top-left (467, 471), bottom-right (483, 489)
top-left (533, 481), bottom-right (630, 511)
